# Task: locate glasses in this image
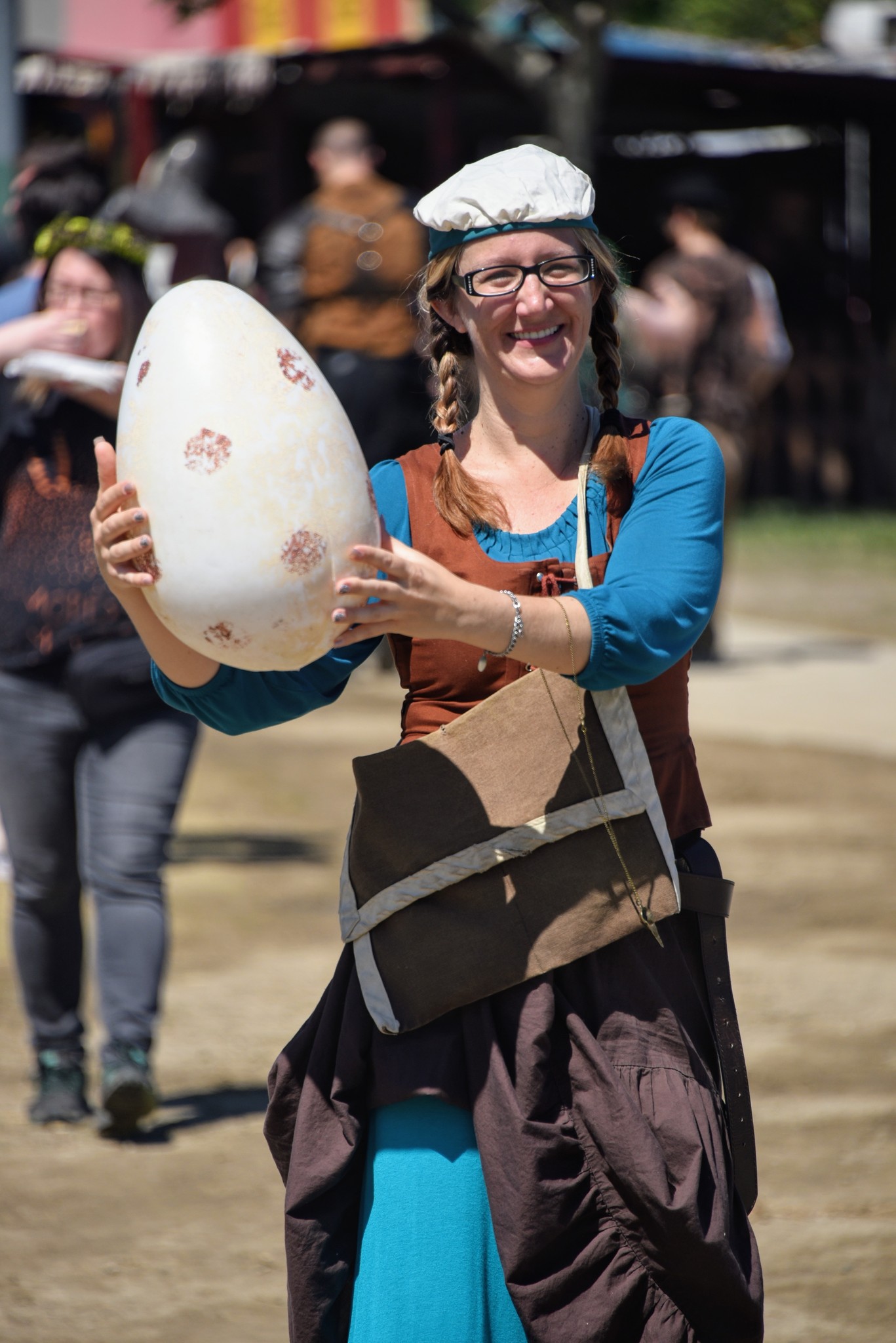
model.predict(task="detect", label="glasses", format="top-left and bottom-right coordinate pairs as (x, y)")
top-left (451, 255), bottom-right (599, 298)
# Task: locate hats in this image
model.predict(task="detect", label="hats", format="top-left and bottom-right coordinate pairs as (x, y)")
top-left (413, 143), bottom-right (598, 259)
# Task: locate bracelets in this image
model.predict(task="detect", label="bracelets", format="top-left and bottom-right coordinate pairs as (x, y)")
top-left (478, 590), bottom-right (524, 672)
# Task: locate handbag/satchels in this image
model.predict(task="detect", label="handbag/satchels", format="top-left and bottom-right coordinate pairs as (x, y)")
top-left (337, 406), bottom-right (682, 1033)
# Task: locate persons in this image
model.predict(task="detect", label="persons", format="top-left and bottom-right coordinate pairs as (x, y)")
top-left (0, 128), bottom-right (267, 329)
top-left (0, 217), bottom-right (200, 1141)
top-left (613, 180), bottom-right (866, 663)
top-left (293, 123), bottom-right (422, 670)
top-left (91, 143), bottom-right (762, 1343)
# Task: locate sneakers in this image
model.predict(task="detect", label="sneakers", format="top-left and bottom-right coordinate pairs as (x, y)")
top-left (98, 1042), bottom-right (154, 1138)
top-left (30, 1046), bottom-right (86, 1125)
